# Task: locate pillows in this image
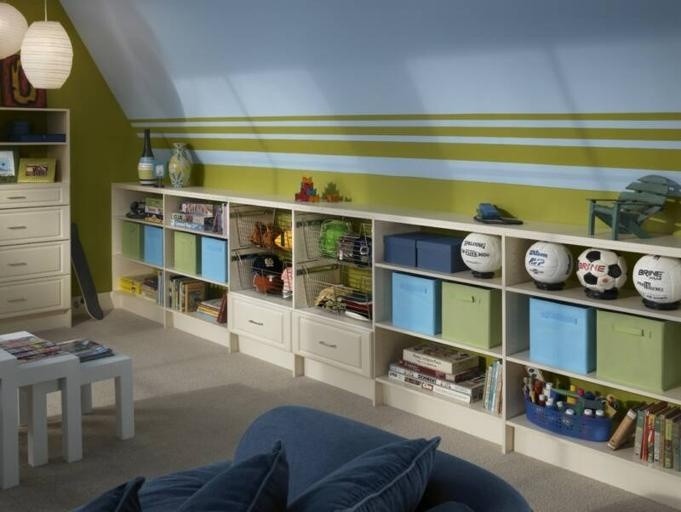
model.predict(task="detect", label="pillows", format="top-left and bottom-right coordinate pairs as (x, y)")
top-left (289, 434), bottom-right (444, 510)
top-left (81, 473), bottom-right (146, 512)
top-left (177, 439), bottom-right (291, 512)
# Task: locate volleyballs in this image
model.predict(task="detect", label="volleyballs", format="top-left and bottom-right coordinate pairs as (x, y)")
top-left (633, 255), bottom-right (681, 304)
top-left (460, 233), bottom-right (501, 272)
top-left (525, 242), bottom-right (573, 283)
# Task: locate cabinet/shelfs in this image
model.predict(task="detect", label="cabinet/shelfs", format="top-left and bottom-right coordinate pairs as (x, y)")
top-left (109, 181), bottom-right (680, 511)
top-left (0, 107), bottom-right (73, 336)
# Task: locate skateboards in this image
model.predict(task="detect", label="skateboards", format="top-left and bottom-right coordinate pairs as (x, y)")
top-left (70, 222), bottom-right (103, 320)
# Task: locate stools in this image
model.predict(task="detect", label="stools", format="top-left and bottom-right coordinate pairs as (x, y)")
top-left (1, 331), bottom-right (136, 491)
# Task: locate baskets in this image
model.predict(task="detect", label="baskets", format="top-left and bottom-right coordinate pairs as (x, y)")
top-left (525, 393), bottom-right (611, 443)
top-left (230, 208), bottom-right (371, 324)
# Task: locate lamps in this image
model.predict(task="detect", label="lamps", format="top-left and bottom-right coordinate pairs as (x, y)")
top-left (20, 0), bottom-right (73, 90)
top-left (0, 2), bottom-right (28, 60)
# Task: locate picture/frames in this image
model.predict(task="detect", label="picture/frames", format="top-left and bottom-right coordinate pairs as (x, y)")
top-left (17, 158), bottom-right (56, 183)
top-left (0, 144), bottom-right (20, 184)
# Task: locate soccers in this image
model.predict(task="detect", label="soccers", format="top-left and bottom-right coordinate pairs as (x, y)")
top-left (576, 248), bottom-right (628, 291)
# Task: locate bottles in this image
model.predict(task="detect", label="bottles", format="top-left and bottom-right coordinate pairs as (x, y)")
top-left (135, 126), bottom-right (157, 187)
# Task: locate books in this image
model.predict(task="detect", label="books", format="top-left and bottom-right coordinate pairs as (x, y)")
top-left (0, 331), bottom-right (61, 368)
top-left (169, 276), bottom-right (227, 324)
top-left (607, 400), bottom-right (681, 472)
top-left (119, 270), bottom-right (164, 306)
top-left (55, 337), bottom-right (112, 363)
top-left (388, 342), bottom-right (503, 415)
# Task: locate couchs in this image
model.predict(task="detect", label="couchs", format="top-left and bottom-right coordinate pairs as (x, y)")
top-left (82, 404), bottom-right (535, 511)
